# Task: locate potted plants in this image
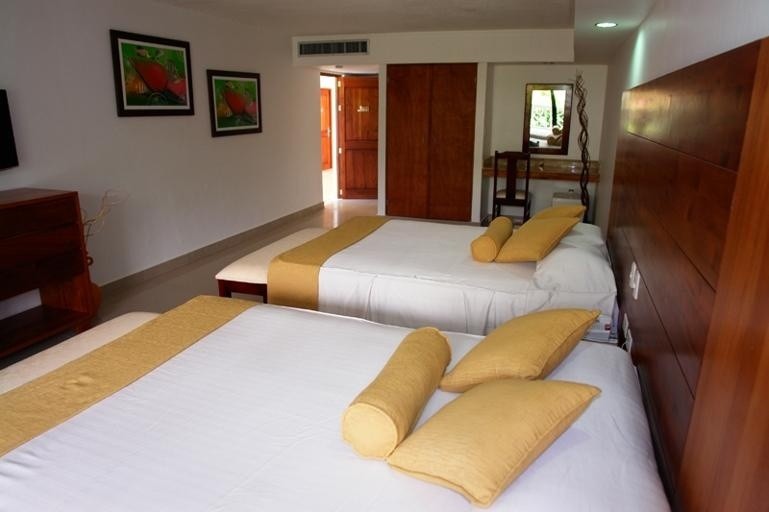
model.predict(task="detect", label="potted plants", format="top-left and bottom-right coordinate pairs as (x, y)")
top-left (81, 189), bottom-right (122, 314)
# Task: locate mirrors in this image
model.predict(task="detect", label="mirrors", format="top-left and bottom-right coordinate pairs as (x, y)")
top-left (522, 82), bottom-right (573, 155)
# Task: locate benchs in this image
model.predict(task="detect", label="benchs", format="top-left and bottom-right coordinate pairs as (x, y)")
top-left (215, 228), bottom-right (331, 304)
top-left (0, 311), bottom-right (161, 399)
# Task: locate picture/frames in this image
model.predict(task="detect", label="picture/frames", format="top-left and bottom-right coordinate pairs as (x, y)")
top-left (206, 69), bottom-right (263, 137)
top-left (109, 29), bottom-right (194, 117)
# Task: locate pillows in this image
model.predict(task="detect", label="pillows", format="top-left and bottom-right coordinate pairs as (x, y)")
top-left (495, 216), bottom-right (580, 263)
top-left (341, 327), bottom-right (451, 461)
top-left (386, 378), bottom-right (601, 510)
top-left (547, 125), bottom-right (563, 146)
top-left (470, 216), bottom-right (512, 263)
top-left (439, 308), bottom-right (602, 392)
top-left (535, 204), bottom-right (587, 218)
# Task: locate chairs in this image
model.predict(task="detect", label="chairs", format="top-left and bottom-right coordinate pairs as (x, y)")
top-left (492, 150), bottom-right (532, 224)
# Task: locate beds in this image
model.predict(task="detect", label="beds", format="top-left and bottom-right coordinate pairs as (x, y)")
top-left (530, 126), bottom-right (552, 146)
top-left (267, 215), bottom-right (618, 336)
top-left (0, 293), bottom-right (672, 511)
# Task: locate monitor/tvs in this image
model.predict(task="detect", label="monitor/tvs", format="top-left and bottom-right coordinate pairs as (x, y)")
top-left (0, 89), bottom-right (19, 172)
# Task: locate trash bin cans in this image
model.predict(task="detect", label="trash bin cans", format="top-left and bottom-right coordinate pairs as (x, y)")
top-left (552, 192), bottom-right (587, 222)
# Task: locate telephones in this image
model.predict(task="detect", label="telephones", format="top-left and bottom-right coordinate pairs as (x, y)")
top-left (584, 315), bottom-right (612, 341)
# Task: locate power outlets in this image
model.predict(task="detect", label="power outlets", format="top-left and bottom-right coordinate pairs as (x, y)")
top-left (625, 329), bottom-right (633, 353)
top-left (621, 312), bottom-right (629, 338)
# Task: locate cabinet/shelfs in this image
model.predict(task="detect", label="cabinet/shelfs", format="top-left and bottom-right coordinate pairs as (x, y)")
top-left (0, 187), bottom-right (98, 359)
top-left (483, 156), bottom-right (600, 183)
